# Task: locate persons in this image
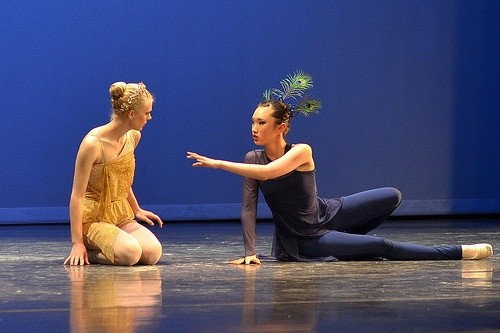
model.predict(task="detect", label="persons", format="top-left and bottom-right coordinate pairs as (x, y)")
top-left (63, 80), bottom-right (165, 264)
top-left (185, 96), bottom-right (494, 265)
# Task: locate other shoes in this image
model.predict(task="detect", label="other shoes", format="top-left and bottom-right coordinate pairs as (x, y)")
top-left (462, 242), bottom-right (493, 259)
